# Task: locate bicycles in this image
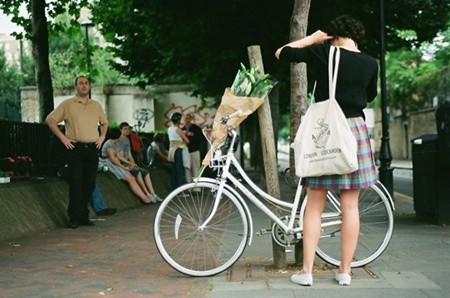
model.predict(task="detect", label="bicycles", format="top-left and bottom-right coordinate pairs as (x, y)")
top-left (152, 109), bottom-right (391, 277)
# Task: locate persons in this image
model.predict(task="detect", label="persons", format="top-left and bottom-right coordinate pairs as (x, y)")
top-left (46, 75), bottom-right (117, 228)
top-left (98, 112), bottom-right (239, 204)
top-left (275, 15), bottom-right (379, 286)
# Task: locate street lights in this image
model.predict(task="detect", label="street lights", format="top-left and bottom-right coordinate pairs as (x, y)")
top-left (80, 22), bottom-right (96, 99)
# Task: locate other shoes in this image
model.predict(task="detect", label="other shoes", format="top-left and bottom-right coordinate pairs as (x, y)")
top-left (69, 220), bottom-right (78, 227)
top-left (153, 194), bottom-right (163, 201)
top-left (80, 220), bottom-right (96, 226)
top-left (334, 271), bottom-right (352, 285)
top-left (97, 207), bottom-right (117, 216)
top-left (147, 194), bottom-right (157, 203)
top-left (291, 271), bottom-right (313, 286)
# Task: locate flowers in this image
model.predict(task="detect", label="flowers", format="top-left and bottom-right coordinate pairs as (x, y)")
top-left (195, 63), bottom-right (279, 178)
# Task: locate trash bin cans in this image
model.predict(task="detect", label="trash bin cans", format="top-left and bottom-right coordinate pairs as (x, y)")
top-left (435, 101), bottom-right (450, 226)
top-left (410, 133), bottom-right (439, 225)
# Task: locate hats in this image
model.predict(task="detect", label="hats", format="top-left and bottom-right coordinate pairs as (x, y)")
top-left (171, 112), bottom-right (182, 124)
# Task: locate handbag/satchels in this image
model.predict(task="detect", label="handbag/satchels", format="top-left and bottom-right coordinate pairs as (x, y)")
top-left (294, 45), bottom-right (359, 178)
